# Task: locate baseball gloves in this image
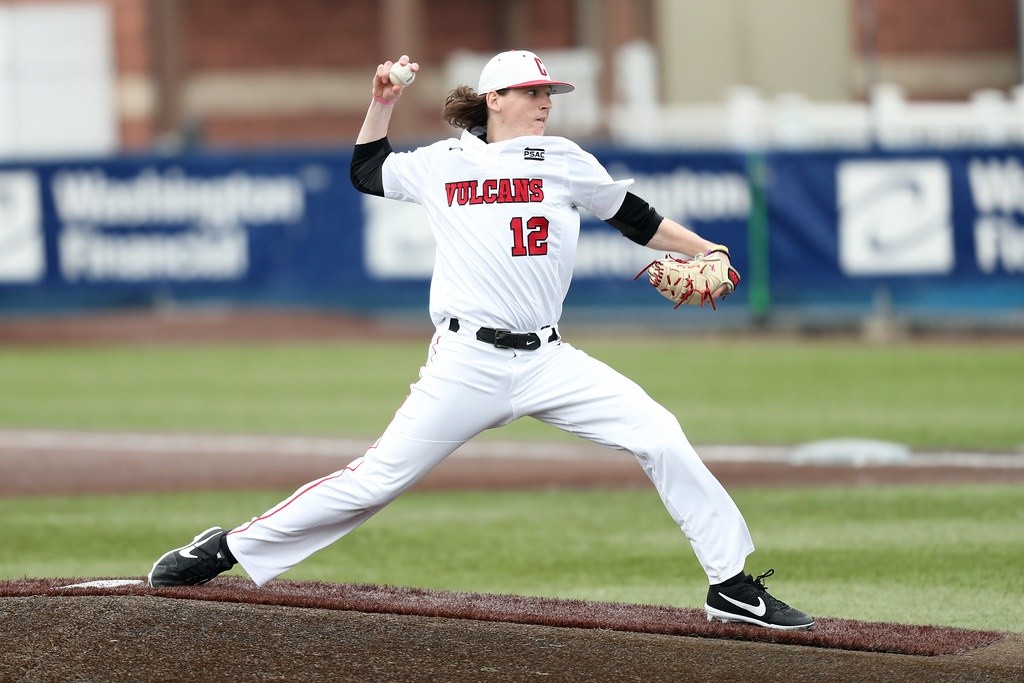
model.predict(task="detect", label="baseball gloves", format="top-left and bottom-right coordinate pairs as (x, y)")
top-left (647, 244), bottom-right (741, 306)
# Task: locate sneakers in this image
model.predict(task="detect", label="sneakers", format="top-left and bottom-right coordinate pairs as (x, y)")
top-left (148, 526), bottom-right (234, 589)
top-left (703, 569), bottom-right (815, 630)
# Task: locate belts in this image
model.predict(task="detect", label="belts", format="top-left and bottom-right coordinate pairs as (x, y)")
top-left (448, 317), bottom-right (559, 351)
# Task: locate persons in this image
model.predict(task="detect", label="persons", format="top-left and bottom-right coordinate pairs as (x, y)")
top-left (149, 44), bottom-right (821, 629)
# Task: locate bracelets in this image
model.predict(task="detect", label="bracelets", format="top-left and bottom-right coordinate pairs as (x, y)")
top-left (372, 92), bottom-right (398, 104)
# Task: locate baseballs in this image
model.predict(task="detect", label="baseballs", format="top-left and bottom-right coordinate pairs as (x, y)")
top-left (387, 60), bottom-right (418, 88)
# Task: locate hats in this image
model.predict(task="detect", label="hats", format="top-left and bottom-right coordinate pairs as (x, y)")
top-left (477, 50), bottom-right (575, 97)
